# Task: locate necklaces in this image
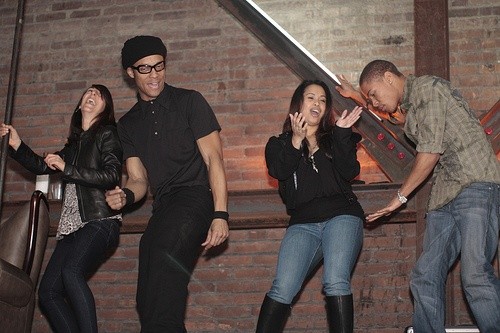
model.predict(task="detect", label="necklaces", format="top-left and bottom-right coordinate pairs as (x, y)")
top-left (308, 143), bottom-right (319, 173)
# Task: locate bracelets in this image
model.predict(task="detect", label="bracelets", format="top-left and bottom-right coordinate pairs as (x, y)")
top-left (212, 211), bottom-right (229, 221)
top-left (397, 190), bottom-right (407, 204)
top-left (122, 188), bottom-right (134, 205)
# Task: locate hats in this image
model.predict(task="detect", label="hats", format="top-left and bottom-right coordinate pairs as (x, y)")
top-left (122, 36), bottom-right (167, 69)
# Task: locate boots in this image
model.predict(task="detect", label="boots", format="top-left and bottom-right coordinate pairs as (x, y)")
top-left (256, 293), bottom-right (292, 333)
top-left (326, 294), bottom-right (353, 333)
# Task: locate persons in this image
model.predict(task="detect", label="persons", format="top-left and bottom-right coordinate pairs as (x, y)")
top-left (334, 60), bottom-right (500, 333)
top-left (255, 80), bottom-right (368, 333)
top-left (104, 35), bottom-right (229, 333)
top-left (0, 84), bottom-right (123, 333)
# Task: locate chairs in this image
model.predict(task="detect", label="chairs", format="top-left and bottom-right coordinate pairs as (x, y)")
top-left (0, 191), bottom-right (50, 333)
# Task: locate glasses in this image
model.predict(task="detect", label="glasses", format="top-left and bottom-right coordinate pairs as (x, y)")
top-left (131, 61), bottom-right (165, 74)
